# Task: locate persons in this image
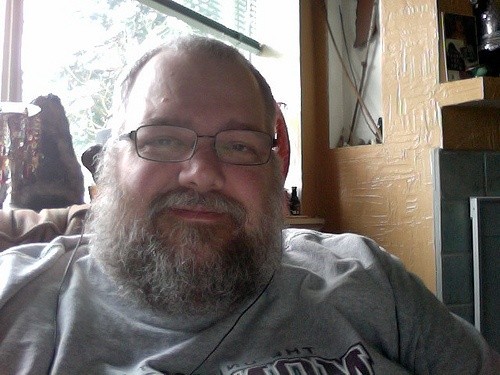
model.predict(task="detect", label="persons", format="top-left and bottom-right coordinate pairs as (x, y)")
top-left (1, 35), bottom-right (500, 375)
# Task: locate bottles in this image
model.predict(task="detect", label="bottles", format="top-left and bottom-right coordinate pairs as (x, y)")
top-left (289, 184), bottom-right (303, 216)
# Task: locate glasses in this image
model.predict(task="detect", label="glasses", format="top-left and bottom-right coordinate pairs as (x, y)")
top-left (119, 123), bottom-right (279, 167)
